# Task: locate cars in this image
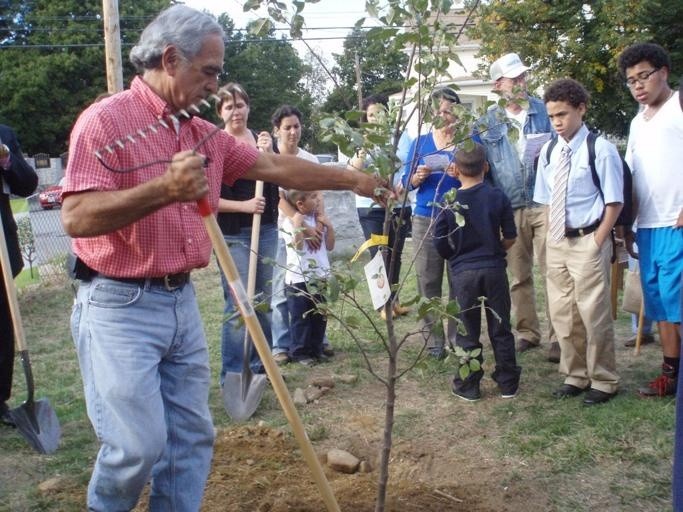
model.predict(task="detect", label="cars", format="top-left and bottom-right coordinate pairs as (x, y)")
top-left (40, 177), bottom-right (67, 211)
top-left (315, 153), bottom-right (336, 164)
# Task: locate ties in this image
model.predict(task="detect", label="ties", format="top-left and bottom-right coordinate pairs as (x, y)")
top-left (548, 144), bottom-right (572, 241)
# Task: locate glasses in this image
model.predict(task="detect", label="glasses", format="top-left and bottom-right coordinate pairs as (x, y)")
top-left (625, 70), bottom-right (660, 88)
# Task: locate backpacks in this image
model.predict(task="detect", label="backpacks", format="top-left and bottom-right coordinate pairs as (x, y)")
top-left (547, 134), bottom-right (639, 233)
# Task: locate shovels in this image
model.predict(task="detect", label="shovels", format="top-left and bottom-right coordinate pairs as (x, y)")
top-left (0, 213), bottom-right (60, 455)
top-left (223, 146), bottom-right (267, 425)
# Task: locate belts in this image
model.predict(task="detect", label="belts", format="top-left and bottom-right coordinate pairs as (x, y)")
top-left (566, 221), bottom-right (600, 238)
top-left (91, 268), bottom-right (190, 291)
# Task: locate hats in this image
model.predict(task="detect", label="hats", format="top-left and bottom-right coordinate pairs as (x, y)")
top-left (489, 53), bottom-right (533, 82)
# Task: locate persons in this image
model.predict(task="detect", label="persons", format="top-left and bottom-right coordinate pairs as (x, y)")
top-left (626, 218), bottom-right (655, 347)
top-left (271, 106), bottom-right (336, 366)
top-left (211, 81), bottom-right (280, 390)
top-left (346, 96), bottom-right (413, 321)
top-left (1, 124), bottom-right (38, 427)
top-left (62, 3), bottom-right (384, 512)
top-left (433, 141), bottom-right (522, 402)
top-left (616, 43), bottom-right (682, 397)
top-left (532, 78), bottom-right (624, 404)
top-left (475, 52), bottom-right (562, 364)
top-left (401, 90), bottom-right (482, 356)
top-left (282, 188), bottom-right (335, 368)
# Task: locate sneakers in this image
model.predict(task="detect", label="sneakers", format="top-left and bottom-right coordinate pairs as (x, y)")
top-left (381, 306), bottom-right (412, 321)
top-left (548, 342), bottom-right (560, 363)
top-left (500, 384), bottom-right (520, 399)
top-left (451, 387), bottom-right (482, 402)
top-left (515, 339), bottom-right (539, 353)
top-left (635, 370), bottom-right (676, 397)
top-left (624, 334), bottom-right (654, 347)
top-left (1, 409), bottom-right (16, 428)
top-left (265, 341), bottom-right (335, 385)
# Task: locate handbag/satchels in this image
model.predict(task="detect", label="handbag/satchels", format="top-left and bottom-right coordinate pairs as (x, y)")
top-left (622, 259), bottom-right (642, 314)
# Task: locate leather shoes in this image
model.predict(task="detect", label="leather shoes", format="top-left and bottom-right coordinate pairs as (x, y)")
top-left (583, 388), bottom-right (618, 405)
top-left (552, 383), bottom-right (591, 398)
top-left (427, 345), bottom-right (446, 361)
